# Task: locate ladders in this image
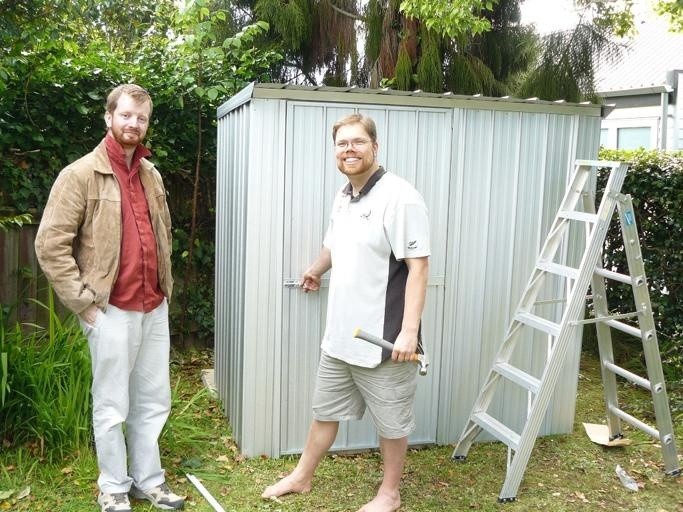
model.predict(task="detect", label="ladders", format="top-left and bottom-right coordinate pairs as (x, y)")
top-left (450, 159), bottom-right (683, 504)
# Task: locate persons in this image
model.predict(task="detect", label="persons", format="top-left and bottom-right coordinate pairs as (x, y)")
top-left (260, 112), bottom-right (433, 512)
top-left (32, 84), bottom-right (190, 512)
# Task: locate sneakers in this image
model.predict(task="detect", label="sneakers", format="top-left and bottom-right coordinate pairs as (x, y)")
top-left (129, 482), bottom-right (184, 511)
top-left (97, 490), bottom-right (132, 512)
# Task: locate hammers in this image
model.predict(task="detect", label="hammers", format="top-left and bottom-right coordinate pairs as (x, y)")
top-left (353, 328), bottom-right (430, 376)
